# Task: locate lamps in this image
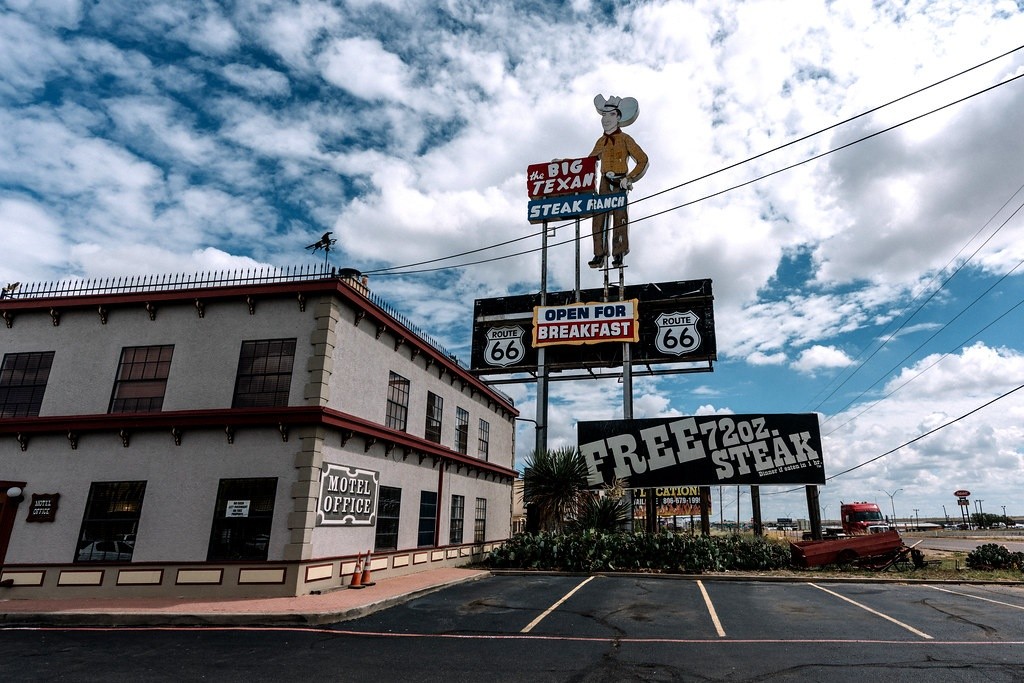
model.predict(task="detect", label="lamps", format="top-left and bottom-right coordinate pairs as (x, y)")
top-left (6, 486), bottom-right (25, 504)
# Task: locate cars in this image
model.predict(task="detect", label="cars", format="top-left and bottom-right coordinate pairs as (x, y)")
top-left (79, 533), bottom-right (135, 562)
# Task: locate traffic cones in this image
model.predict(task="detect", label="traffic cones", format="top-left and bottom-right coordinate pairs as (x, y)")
top-left (347, 549), bottom-right (376, 590)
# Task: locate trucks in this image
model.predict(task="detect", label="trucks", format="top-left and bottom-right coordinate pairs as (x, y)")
top-left (827, 503), bottom-right (886, 535)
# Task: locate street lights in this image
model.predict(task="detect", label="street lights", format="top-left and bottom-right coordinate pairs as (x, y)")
top-left (1001, 505), bottom-right (1008, 528)
top-left (912, 508), bottom-right (919, 531)
top-left (820, 503), bottom-right (832, 525)
top-left (878, 489), bottom-right (904, 530)
